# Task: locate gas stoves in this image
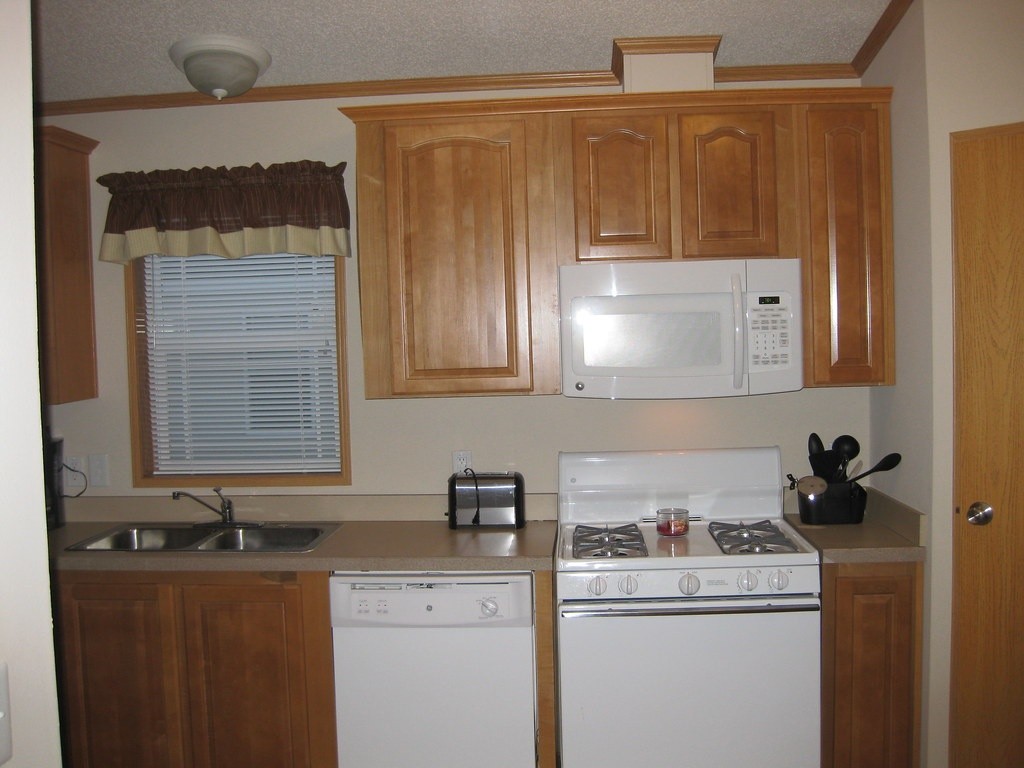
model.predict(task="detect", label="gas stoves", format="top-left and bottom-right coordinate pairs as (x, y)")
top-left (557, 446), bottom-right (820, 598)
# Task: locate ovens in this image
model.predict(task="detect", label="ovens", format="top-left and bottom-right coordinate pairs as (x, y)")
top-left (557, 597), bottom-right (820, 767)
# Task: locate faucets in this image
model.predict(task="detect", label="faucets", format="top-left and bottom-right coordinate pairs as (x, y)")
top-left (173, 488), bottom-right (233, 522)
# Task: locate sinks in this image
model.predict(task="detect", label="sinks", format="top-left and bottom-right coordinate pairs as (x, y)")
top-left (198, 526), bottom-right (324, 555)
top-left (81, 526), bottom-right (209, 555)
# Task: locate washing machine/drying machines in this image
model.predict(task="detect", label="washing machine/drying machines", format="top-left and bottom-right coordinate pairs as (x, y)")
top-left (330, 571), bottom-right (539, 767)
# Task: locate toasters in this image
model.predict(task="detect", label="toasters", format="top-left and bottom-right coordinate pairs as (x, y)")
top-left (444, 470), bottom-right (524, 530)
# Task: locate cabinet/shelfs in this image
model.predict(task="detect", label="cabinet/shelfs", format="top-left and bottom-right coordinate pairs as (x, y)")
top-left (337, 87), bottom-right (897, 400)
top-left (51, 562), bottom-right (926, 768)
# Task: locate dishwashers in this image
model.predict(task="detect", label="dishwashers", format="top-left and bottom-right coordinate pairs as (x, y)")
top-left (329, 569), bottom-right (538, 768)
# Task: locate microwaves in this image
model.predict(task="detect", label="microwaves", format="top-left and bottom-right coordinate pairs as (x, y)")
top-left (559, 259), bottom-right (803, 400)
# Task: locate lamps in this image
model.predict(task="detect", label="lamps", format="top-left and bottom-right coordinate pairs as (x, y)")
top-left (167, 35), bottom-right (272, 103)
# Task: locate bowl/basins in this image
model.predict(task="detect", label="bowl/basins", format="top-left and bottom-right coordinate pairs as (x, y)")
top-left (656, 508), bottom-right (689, 536)
